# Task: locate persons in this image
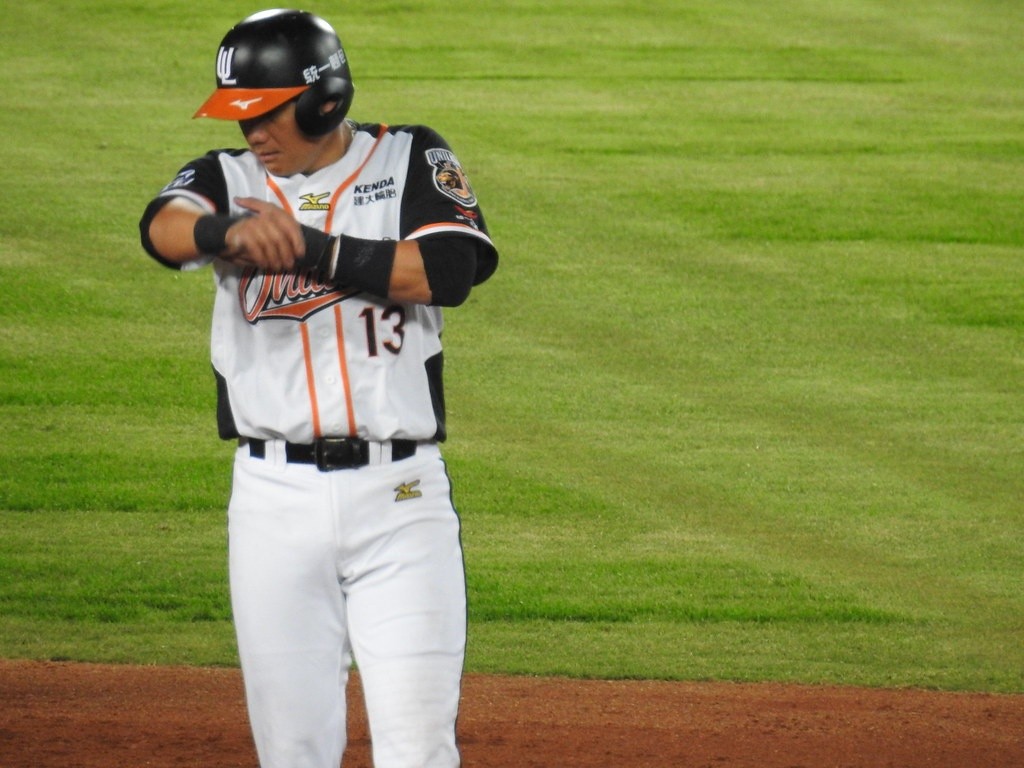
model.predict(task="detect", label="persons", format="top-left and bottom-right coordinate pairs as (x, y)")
top-left (139, 7), bottom-right (499, 768)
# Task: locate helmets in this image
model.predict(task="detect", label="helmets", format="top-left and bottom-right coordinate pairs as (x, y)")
top-left (192, 7), bottom-right (356, 137)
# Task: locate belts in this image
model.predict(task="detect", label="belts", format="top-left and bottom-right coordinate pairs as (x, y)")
top-left (248, 435), bottom-right (416, 471)
